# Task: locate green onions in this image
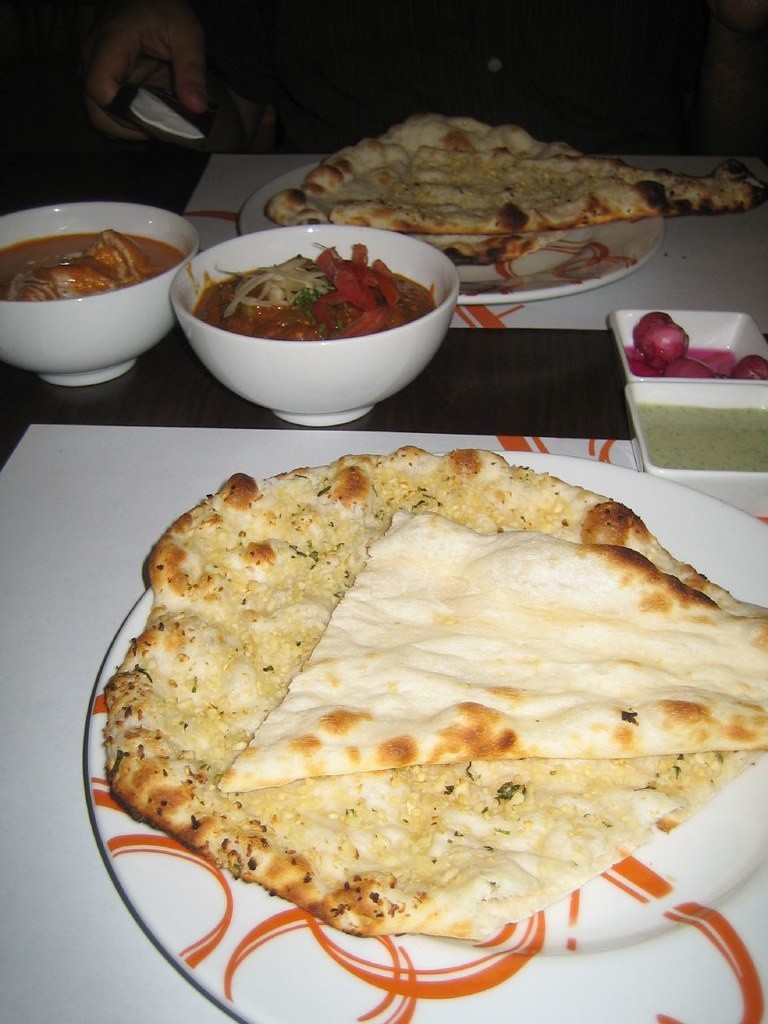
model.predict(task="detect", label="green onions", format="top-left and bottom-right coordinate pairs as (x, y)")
top-left (293, 282), bottom-right (353, 337)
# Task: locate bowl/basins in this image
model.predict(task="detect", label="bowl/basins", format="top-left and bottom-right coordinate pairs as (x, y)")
top-left (0, 201), bottom-right (200, 387)
top-left (169, 224), bottom-right (460, 427)
top-left (608, 309), bottom-right (768, 381)
top-left (624, 382), bottom-right (768, 518)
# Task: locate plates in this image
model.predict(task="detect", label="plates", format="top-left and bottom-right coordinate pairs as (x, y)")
top-left (81, 449), bottom-right (768, 1024)
top-left (237, 161), bottom-right (666, 306)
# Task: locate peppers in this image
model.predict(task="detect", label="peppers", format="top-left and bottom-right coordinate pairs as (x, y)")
top-left (316, 244), bottom-right (399, 337)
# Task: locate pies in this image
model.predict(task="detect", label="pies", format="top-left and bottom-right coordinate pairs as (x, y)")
top-left (105, 443), bottom-right (768, 938)
top-left (217, 509), bottom-right (768, 791)
top-left (263, 109), bottom-right (586, 266)
top-left (330, 143), bottom-right (768, 234)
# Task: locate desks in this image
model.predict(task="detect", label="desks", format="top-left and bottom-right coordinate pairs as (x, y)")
top-left (0, 152), bottom-right (768, 1024)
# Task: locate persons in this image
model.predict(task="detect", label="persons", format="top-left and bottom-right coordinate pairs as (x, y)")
top-left (82, 0), bottom-right (768, 156)
